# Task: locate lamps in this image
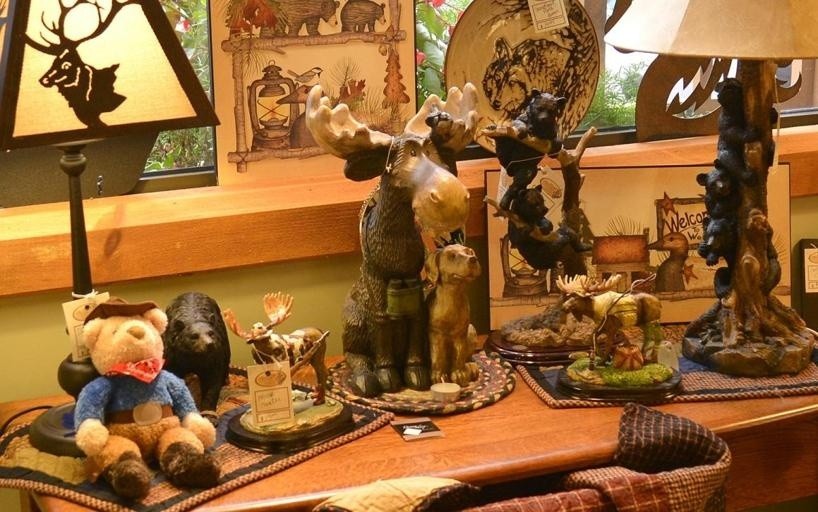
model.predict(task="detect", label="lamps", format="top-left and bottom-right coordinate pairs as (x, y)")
top-left (1, 0), bottom-right (221, 457)
top-left (600, 0), bottom-right (818, 376)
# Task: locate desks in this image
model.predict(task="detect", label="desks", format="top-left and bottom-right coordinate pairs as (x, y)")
top-left (0, 364), bottom-right (816, 511)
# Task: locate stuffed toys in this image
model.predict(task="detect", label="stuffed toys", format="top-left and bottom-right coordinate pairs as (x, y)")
top-left (73, 298), bottom-right (220, 499)
